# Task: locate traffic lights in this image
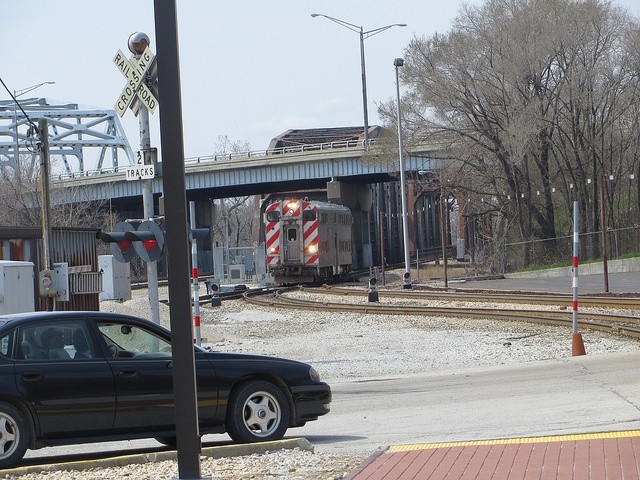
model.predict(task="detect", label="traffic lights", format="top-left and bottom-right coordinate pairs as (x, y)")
top-left (100, 222), bottom-right (136, 263)
top-left (125, 221), bottom-right (165, 262)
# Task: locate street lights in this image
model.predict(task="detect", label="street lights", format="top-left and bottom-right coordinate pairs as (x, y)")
top-left (311, 14), bottom-right (407, 148)
top-left (14, 82), bottom-right (56, 185)
top-left (393, 58), bottom-right (410, 273)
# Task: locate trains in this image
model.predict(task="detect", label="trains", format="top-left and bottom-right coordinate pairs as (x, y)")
top-left (265, 196), bottom-right (354, 285)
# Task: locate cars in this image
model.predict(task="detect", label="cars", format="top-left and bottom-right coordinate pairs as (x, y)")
top-left (0, 311), bottom-right (332, 469)
